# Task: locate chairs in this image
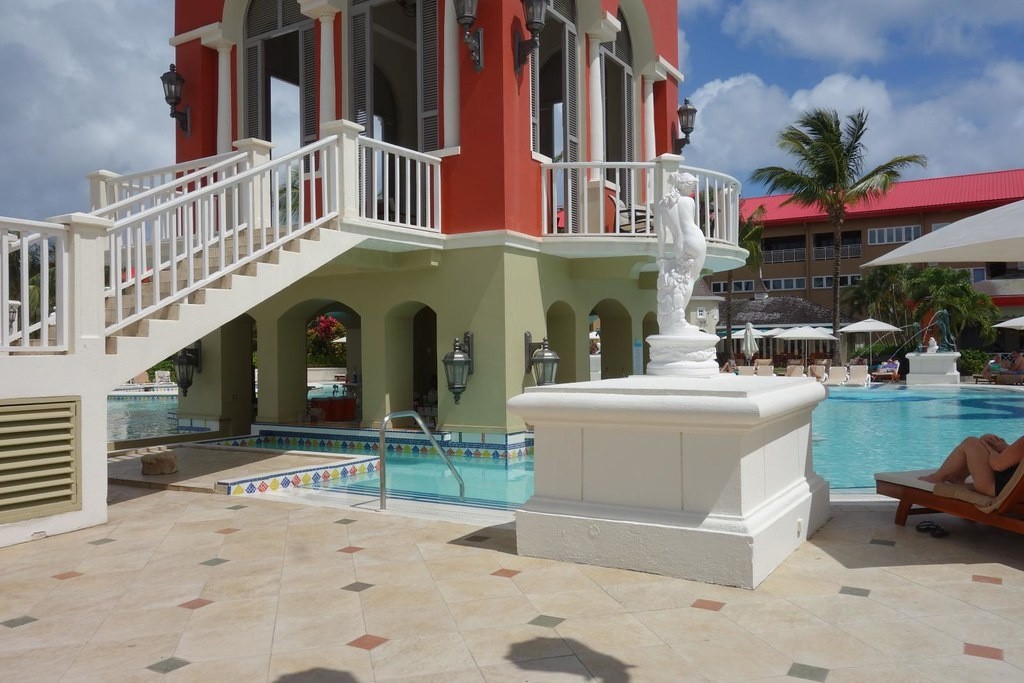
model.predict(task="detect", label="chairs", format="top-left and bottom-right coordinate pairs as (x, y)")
top-left (737, 359), bottom-right (902, 387)
top-left (874, 457), bottom-right (1024, 534)
top-left (324, 400), bottom-right (356, 421)
top-left (972, 360), bottom-right (1024, 386)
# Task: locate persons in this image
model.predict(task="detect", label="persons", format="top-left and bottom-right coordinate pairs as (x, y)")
top-left (882, 359), bottom-right (900, 383)
top-left (721, 356), bottom-right (736, 372)
top-left (984, 350), bottom-right (1024, 385)
top-left (917, 434), bottom-right (1024, 496)
top-left (657, 172), bottom-right (706, 337)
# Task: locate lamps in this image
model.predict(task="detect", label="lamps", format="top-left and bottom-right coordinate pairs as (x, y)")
top-left (523, 331), bottom-right (560, 386)
top-left (173, 340), bottom-right (201, 398)
top-left (452, 0), bottom-right (483, 70)
top-left (674, 98), bottom-right (697, 157)
top-left (513, 0), bottom-right (549, 72)
top-left (8, 305), bottom-right (16, 328)
top-left (442, 331), bottom-right (474, 406)
top-left (160, 63), bottom-right (189, 137)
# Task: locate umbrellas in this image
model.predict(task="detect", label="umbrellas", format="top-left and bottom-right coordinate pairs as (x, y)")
top-left (721, 329), bottom-right (765, 339)
top-left (763, 326), bottom-right (840, 376)
top-left (836, 316), bottom-right (904, 366)
top-left (858, 197), bottom-right (1024, 267)
top-left (740, 322), bottom-right (759, 367)
top-left (990, 316), bottom-right (1024, 330)
